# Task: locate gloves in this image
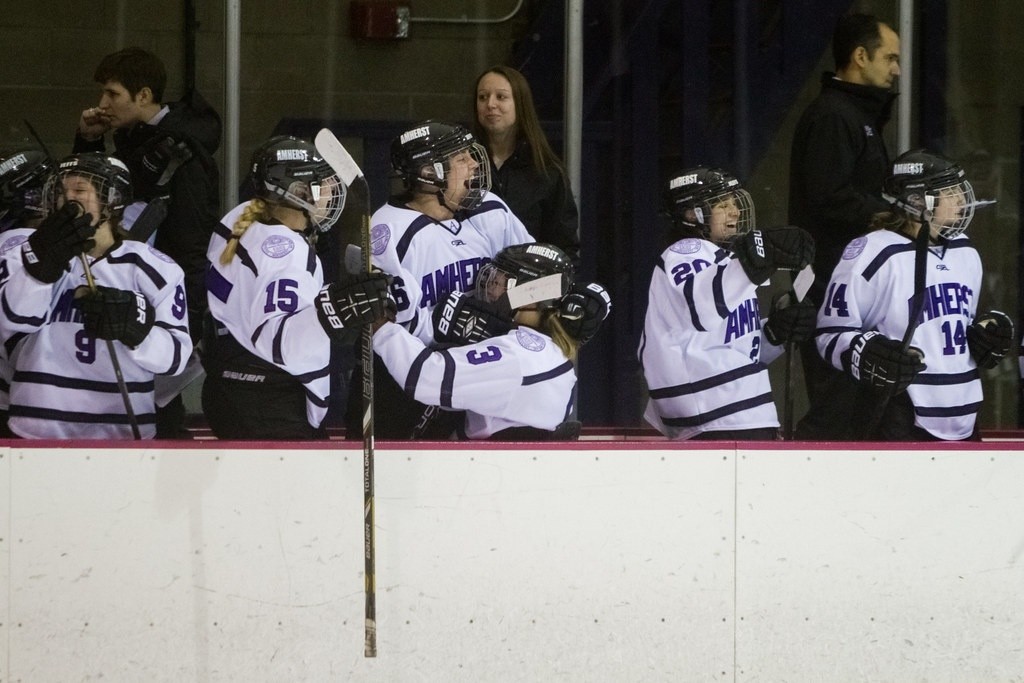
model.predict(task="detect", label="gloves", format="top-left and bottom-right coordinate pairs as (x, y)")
top-left (315, 272), bottom-right (398, 341)
top-left (69, 283), bottom-right (156, 347)
top-left (130, 139), bottom-right (192, 191)
top-left (765, 297), bottom-right (815, 345)
top-left (968, 309), bottom-right (1018, 370)
top-left (21, 205), bottom-right (96, 283)
top-left (848, 331), bottom-right (927, 394)
top-left (731, 227), bottom-right (815, 284)
top-left (433, 289), bottom-right (512, 347)
top-left (557, 280), bottom-right (612, 341)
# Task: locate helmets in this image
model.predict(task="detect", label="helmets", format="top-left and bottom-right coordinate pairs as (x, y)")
top-left (43, 152), bottom-right (136, 233)
top-left (668, 166), bottom-right (756, 244)
top-left (474, 243), bottom-right (576, 321)
top-left (0, 144), bottom-right (54, 208)
top-left (882, 148), bottom-right (976, 241)
top-left (248, 136), bottom-right (347, 233)
top-left (389, 120), bottom-right (492, 213)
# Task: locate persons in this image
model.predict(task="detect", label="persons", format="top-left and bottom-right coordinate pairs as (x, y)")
top-left (815, 146), bottom-right (1024, 441)
top-left (787, 14), bottom-right (903, 441)
top-left (636, 159), bottom-right (814, 441)
top-left (343, 116), bottom-right (535, 441)
top-left (200, 133), bottom-right (398, 443)
top-left (70, 49), bottom-right (219, 441)
top-left (369, 242), bottom-right (578, 441)
top-left (463, 64), bottom-right (579, 267)
top-left (0, 150), bottom-right (195, 439)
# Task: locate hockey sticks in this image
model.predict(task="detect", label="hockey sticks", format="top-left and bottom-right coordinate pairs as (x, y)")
top-left (312, 126), bottom-right (382, 662)
top-left (784, 263), bottom-right (818, 439)
top-left (864, 220), bottom-right (932, 440)
top-left (16, 114), bottom-right (141, 440)
